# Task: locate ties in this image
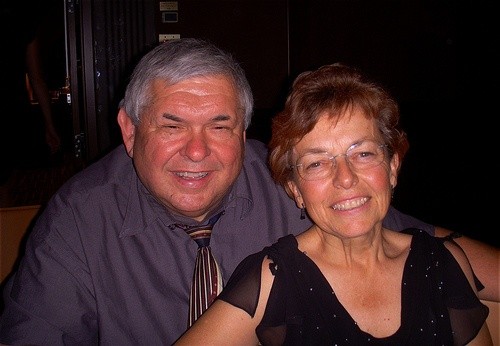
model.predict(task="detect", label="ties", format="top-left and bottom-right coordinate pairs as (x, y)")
top-left (173, 211), bottom-right (224, 327)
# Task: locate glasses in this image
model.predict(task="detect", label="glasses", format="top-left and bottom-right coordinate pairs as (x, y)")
top-left (292, 140), bottom-right (390, 181)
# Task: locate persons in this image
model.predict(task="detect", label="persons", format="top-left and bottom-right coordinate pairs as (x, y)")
top-left (24, 19), bottom-right (75, 166)
top-left (0, 40), bottom-right (500, 346)
top-left (173, 64), bottom-right (493, 346)
